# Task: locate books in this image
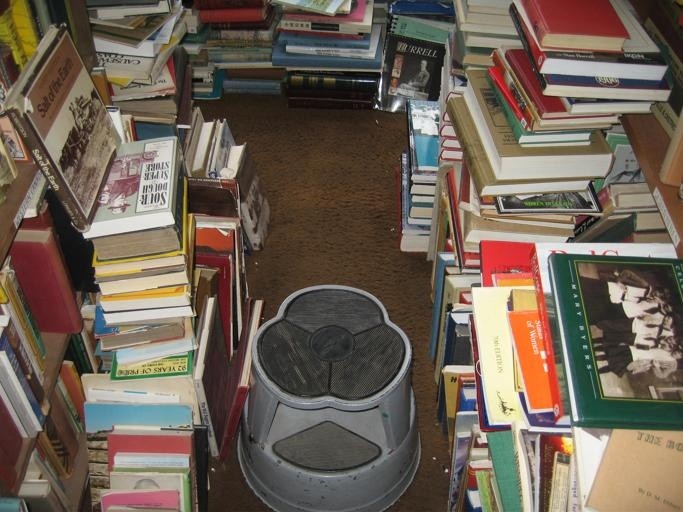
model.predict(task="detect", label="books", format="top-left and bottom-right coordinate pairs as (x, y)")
top-left (1, 1), bottom-right (275, 512)
top-left (278, 0), bottom-right (400, 113)
top-left (399, 1), bottom-right (681, 511)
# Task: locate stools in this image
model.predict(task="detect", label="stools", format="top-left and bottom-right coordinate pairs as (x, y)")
top-left (236, 284), bottom-right (421, 512)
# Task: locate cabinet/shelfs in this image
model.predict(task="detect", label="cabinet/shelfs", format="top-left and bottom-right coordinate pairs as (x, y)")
top-left (0, 0), bottom-right (112, 511)
